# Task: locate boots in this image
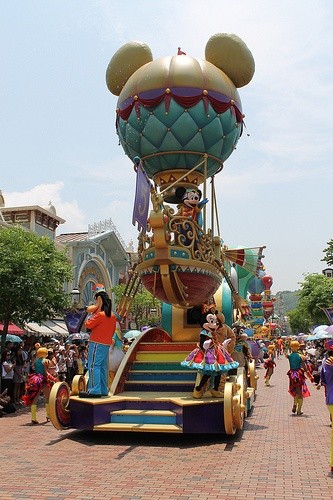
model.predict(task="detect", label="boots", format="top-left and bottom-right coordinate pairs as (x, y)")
top-left (45, 403), bottom-right (51, 423)
top-left (31, 404), bottom-right (39, 425)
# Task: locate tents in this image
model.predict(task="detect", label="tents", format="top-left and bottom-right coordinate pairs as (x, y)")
top-left (0, 319), bottom-right (86, 336)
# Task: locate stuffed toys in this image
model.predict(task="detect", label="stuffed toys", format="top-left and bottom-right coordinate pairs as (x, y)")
top-left (80, 284), bottom-right (116, 398)
top-left (170, 186), bottom-right (209, 254)
top-left (181, 308), bottom-right (239, 398)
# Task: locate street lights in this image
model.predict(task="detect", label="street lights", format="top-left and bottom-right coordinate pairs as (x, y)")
top-left (70, 288), bottom-right (81, 308)
top-left (150, 306), bottom-right (157, 324)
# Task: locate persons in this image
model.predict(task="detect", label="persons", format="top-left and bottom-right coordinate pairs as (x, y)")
top-left (286, 341), bottom-right (311, 415)
top-left (264, 345), bottom-right (276, 386)
top-left (0, 337), bottom-right (89, 417)
top-left (21, 347), bottom-right (59, 424)
top-left (321, 340), bottom-right (333, 427)
top-left (108, 311), bottom-right (125, 392)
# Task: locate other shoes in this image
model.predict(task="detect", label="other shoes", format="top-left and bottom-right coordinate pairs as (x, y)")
top-left (297, 398), bottom-right (304, 415)
top-left (292, 396), bottom-right (297, 413)
